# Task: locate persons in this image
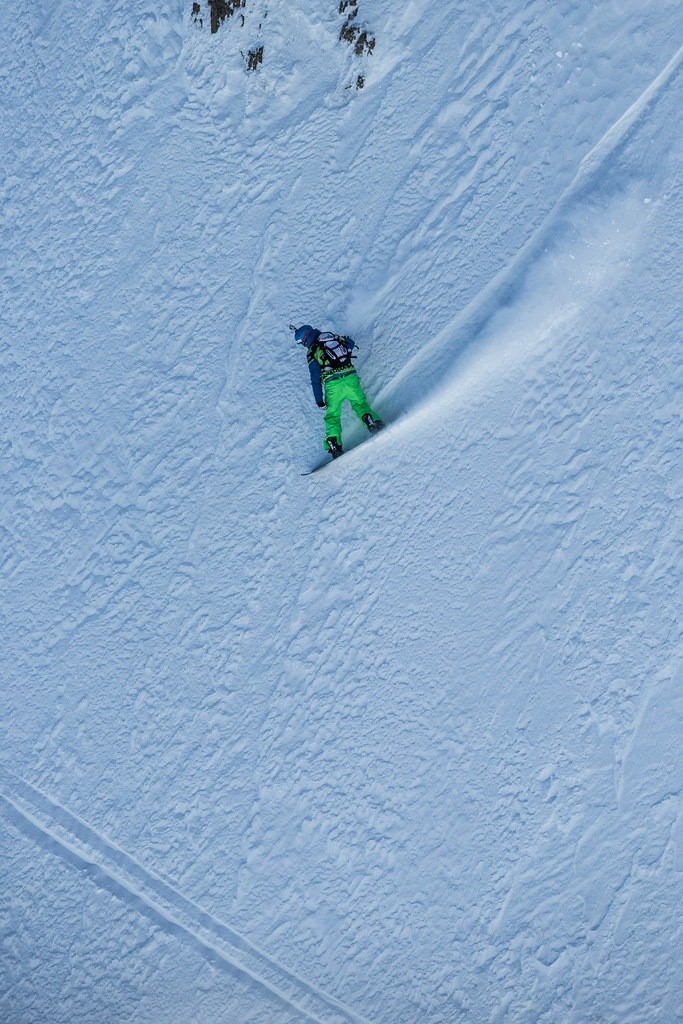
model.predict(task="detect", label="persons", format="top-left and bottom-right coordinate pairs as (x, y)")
top-left (293, 322), bottom-right (386, 465)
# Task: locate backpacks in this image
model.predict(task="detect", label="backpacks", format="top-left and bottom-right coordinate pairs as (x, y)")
top-left (318, 331), bottom-right (355, 369)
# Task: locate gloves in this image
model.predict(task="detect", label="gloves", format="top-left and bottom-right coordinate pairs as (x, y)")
top-left (317, 402), bottom-right (328, 410)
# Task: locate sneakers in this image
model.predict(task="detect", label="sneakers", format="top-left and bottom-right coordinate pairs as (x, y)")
top-left (326, 436), bottom-right (345, 460)
top-left (363, 413), bottom-right (385, 434)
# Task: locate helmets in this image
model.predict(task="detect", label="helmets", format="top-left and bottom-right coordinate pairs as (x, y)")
top-left (294, 325), bottom-right (314, 345)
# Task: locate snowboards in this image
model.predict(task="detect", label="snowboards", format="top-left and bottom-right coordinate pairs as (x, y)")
top-left (299, 420), bottom-right (390, 478)
